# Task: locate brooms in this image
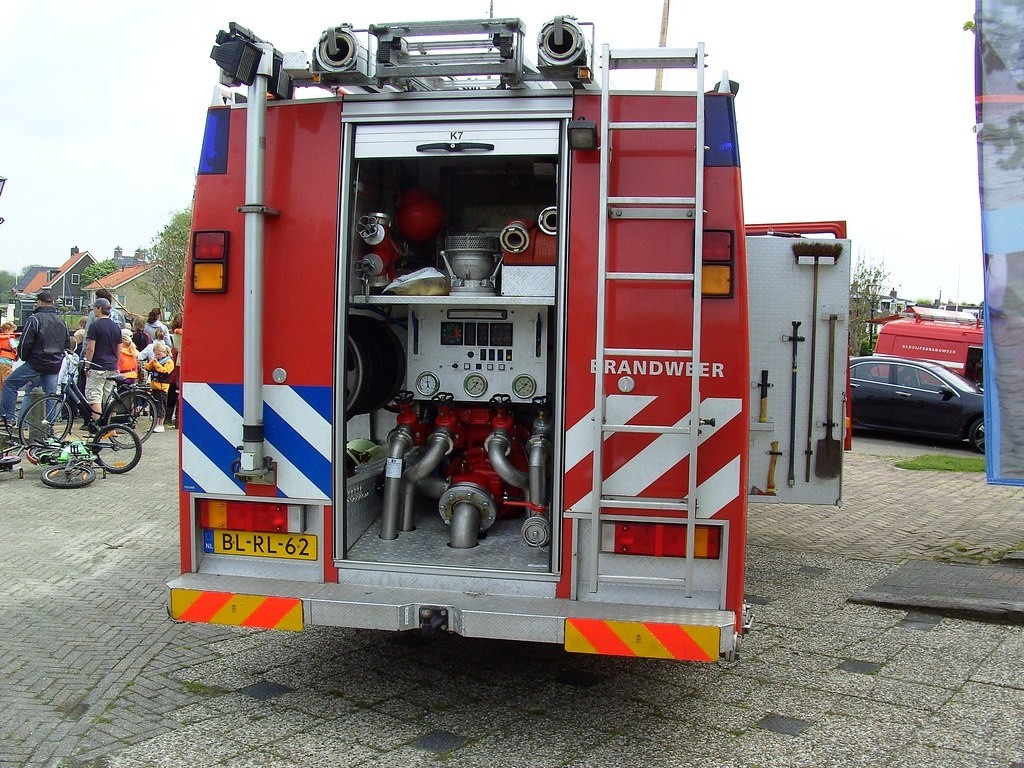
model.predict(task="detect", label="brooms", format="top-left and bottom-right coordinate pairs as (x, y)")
top-left (791, 242), bottom-right (845, 483)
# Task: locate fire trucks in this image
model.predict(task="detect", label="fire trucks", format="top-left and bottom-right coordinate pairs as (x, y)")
top-left (163, 6), bottom-right (857, 667)
top-left (869, 305), bottom-right (986, 390)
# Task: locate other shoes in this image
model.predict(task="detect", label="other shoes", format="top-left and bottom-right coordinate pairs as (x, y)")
top-left (83, 434), bottom-right (95, 438)
top-left (0, 420), bottom-right (53, 431)
top-left (80, 423), bottom-right (90, 430)
top-left (105, 410), bottom-right (148, 433)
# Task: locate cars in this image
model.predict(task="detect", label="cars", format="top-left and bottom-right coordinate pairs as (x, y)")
top-left (846, 355), bottom-right (985, 453)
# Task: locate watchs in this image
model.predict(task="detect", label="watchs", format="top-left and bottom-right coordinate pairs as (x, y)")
top-left (83, 367), bottom-right (89, 372)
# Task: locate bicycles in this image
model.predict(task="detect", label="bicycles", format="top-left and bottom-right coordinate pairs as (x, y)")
top-left (0, 348), bottom-right (158, 490)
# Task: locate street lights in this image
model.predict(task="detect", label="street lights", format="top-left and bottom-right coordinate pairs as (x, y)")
top-left (866, 279), bottom-right (881, 353)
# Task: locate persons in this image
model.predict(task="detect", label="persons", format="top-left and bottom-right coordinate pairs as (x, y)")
top-left (0, 290), bottom-right (182, 437)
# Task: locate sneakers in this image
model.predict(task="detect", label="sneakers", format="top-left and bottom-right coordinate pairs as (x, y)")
top-left (153, 424), bottom-right (165, 433)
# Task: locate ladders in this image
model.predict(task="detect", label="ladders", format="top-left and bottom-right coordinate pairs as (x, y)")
top-left (373, 20), bottom-right (526, 76)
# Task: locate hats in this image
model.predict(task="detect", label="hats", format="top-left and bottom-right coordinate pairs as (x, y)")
top-left (94, 298), bottom-right (115, 315)
top-left (37, 292), bottom-right (53, 302)
